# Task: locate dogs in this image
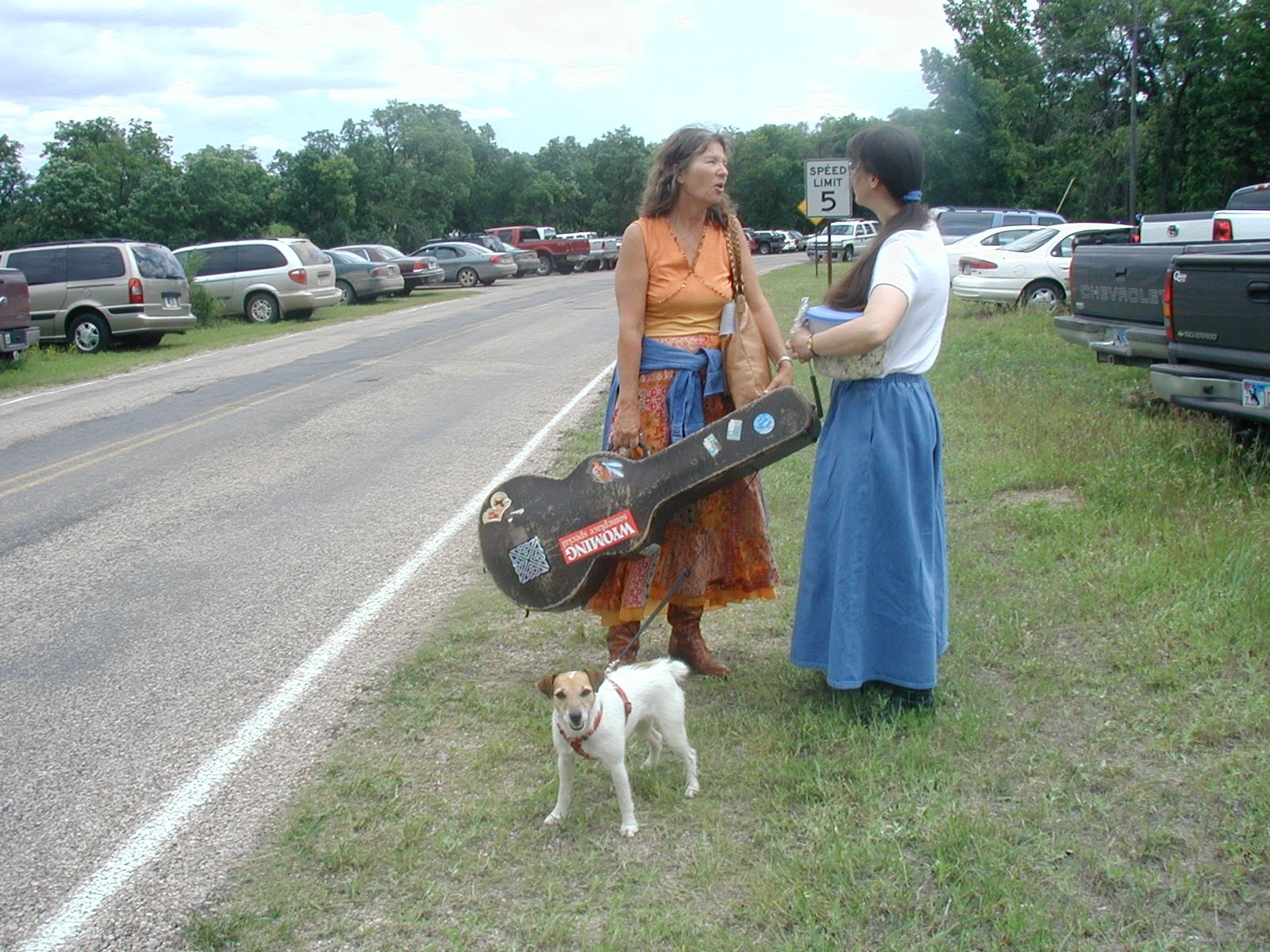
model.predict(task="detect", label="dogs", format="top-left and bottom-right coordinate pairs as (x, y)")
top-left (537, 655), bottom-right (699, 838)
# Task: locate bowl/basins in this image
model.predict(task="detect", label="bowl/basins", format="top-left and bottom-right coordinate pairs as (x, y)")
top-left (807, 306), bottom-right (888, 381)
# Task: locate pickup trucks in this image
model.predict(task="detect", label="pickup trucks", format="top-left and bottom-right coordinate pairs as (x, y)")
top-left (484, 227), bottom-right (622, 276)
top-left (1054, 184), bottom-right (1270, 417)
top-left (0, 268), bottom-right (40, 360)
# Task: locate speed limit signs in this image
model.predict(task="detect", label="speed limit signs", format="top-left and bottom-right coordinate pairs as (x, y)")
top-left (806, 156), bottom-right (851, 219)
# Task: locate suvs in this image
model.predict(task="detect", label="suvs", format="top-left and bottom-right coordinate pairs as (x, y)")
top-left (170, 238), bottom-right (344, 325)
top-left (927, 206), bottom-right (1068, 246)
top-left (0, 238), bottom-right (197, 353)
top-left (807, 219), bottom-right (879, 262)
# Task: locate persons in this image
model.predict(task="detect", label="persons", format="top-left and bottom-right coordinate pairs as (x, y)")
top-left (583, 124), bottom-right (795, 679)
top-left (785, 128), bottom-right (951, 695)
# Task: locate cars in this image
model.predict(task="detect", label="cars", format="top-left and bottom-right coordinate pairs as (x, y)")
top-left (322, 250), bottom-right (405, 306)
top-left (331, 245), bottom-right (446, 297)
top-left (943, 223), bottom-right (1135, 314)
top-left (406, 230), bottom-right (541, 287)
top-left (743, 227), bottom-right (814, 255)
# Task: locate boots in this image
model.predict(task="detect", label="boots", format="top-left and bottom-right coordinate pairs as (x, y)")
top-left (666, 601), bottom-right (727, 676)
top-left (606, 620), bottom-right (641, 671)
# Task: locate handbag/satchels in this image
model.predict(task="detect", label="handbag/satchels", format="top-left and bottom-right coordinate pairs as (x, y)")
top-left (717, 213), bottom-right (771, 410)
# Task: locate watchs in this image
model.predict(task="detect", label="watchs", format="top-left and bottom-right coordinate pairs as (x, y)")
top-left (806, 332), bottom-right (820, 360)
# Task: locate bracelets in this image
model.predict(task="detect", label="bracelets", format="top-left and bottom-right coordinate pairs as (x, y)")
top-left (776, 355), bottom-right (794, 372)
